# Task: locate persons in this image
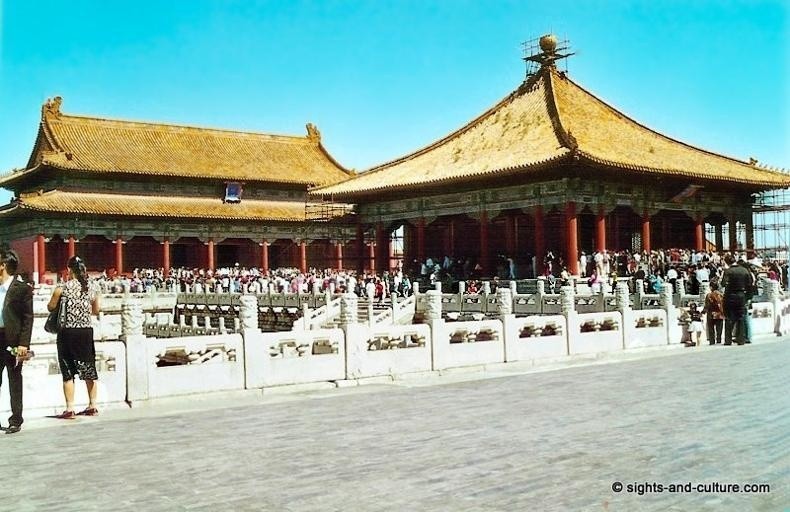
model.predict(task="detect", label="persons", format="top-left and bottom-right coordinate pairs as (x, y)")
top-left (100, 248), bottom-right (789, 293)
top-left (0, 247), bottom-right (33, 434)
top-left (48, 256), bottom-right (102, 419)
top-left (703, 281), bottom-right (723, 344)
top-left (738, 261), bottom-right (756, 342)
top-left (687, 302), bottom-right (703, 347)
top-left (722, 255), bottom-right (751, 346)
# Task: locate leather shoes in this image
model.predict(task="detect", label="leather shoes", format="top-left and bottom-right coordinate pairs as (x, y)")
top-left (5, 424), bottom-right (21, 434)
top-left (76, 407), bottom-right (98, 416)
top-left (57, 410), bottom-right (75, 419)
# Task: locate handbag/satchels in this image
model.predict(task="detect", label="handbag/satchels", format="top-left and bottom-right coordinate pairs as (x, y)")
top-left (44, 296), bottom-right (69, 334)
